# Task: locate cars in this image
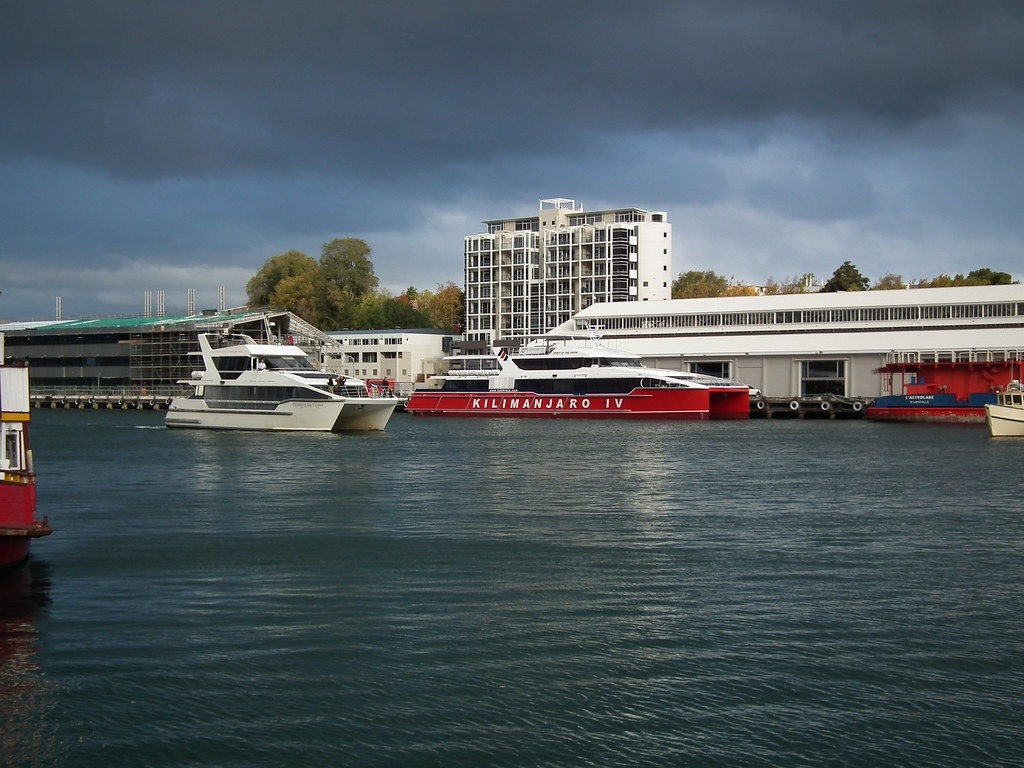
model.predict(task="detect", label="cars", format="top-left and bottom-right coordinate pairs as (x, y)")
top-left (747, 384), bottom-right (763, 398)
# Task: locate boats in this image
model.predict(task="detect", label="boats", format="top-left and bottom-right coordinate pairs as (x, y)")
top-left (864, 391), bottom-right (996, 425)
top-left (985, 379), bottom-right (1024, 440)
top-left (0, 362), bottom-right (53, 567)
top-left (163, 330), bottom-right (402, 434)
top-left (402, 322), bottom-right (752, 423)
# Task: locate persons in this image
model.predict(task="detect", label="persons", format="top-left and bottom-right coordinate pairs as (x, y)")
top-left (327, 375), bottom-right (347, 397)
top-left (288, 334), bottom-right (294, 345)
top-left (218, 331), bottom-right (234, 350)
top-left (367, 376), bottom-right (395, 398)
top-left (258, 358), bottom-right (266, 371)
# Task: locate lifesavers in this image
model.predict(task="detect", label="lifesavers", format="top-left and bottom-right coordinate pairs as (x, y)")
top-left (821, 401), bottom-right (830, 410)
top-left (852, 401), bottom-right (862, 412)
top-left (789, 400), bottom-right (799, 410)
top-left (757, 401), bottom-right (765, 409)
